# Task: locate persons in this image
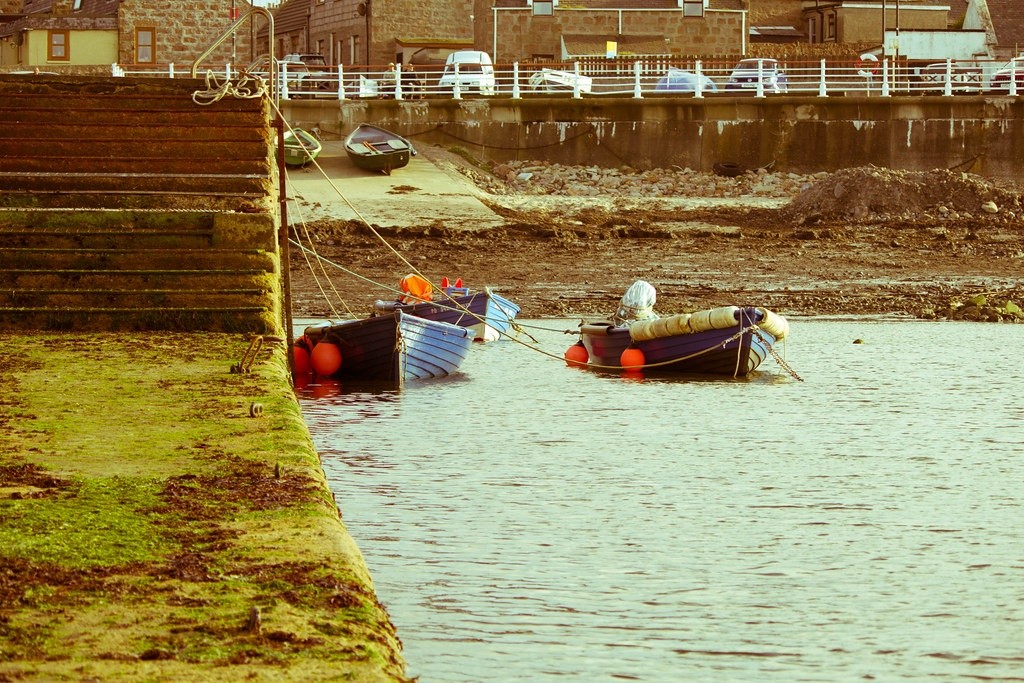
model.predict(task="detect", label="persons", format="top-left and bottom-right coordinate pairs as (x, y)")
top-left (402, 63), bottom-right (423, 99)
top-left (383, 63), bottom-right (402, 99)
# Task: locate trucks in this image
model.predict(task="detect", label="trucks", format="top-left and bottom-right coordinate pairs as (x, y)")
top-left (438, 51), bottom-right (495, 96)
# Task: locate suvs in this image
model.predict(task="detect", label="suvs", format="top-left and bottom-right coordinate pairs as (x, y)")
top-left (284, 51), bottom-right (331, 89)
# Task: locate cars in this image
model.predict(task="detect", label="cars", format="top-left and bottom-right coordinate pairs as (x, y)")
top-left (921, 64), bottom-right (969, 93)
top-left (256, 61), bottom-right (310, 98)
top-left (725, 58), bottom-right (788, 94)
top-left (990, 57), bottom-right (1024, 95)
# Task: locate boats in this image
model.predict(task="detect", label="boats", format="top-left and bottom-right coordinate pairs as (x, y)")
top-left (273, 128), bottom-right (321, 165)
top-left (580, 306), bottom-right (789, 375)
top-left (344, 122), bottom-right (417, 176)
top-left (304, 309), bottom-right (476, 386)
top-left (376, 286), bottom-right (521, 343)
top-left (528, 68), bottom-right (592, 95)
